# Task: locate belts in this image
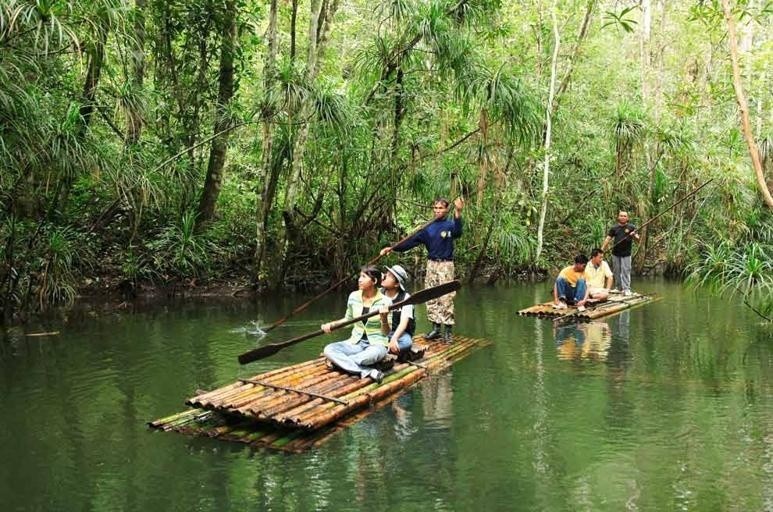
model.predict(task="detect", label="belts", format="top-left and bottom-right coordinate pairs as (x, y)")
top-left (427, 254), bottom-right (453, 262)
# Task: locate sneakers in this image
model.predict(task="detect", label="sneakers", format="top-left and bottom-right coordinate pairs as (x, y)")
top-left (608, 288), bottom-right (622, 294)
top-left (551, 301), bottom-right (568, 310)
top-left (623, 288), bottom-right (631, 296)
top-left (326, 361), bottom-right (337, 370)
top-left (369, 368), bottom-right (386, 385)
top-left (577, 305), bottom-right (587, 312)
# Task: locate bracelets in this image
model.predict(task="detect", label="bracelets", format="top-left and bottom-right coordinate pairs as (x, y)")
top-left (381, 321), bottom-right (388, 324)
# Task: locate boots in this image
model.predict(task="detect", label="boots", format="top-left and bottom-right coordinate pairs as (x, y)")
top-left (423, 321), bottom-right (442, 340)
top-left (444, 324), bottom-right (454, 344)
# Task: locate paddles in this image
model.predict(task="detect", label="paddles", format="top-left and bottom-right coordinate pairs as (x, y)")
top-left (238, 281), bottom-right (462, 365)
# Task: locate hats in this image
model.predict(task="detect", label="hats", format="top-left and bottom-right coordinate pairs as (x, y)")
top-left (382, 264), bottom-right (409, 292)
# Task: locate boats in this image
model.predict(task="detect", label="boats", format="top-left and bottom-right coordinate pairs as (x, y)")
top-left (516, 287), bottom-right (657, 322)
top-left (146, 329), bottom-right (495, 460)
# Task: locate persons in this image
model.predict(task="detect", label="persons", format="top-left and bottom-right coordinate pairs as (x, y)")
top-left (584, 249), bottom-right (614, 302)
top-left (552, 254), bottom-right (590, 312)
top-left (600, 210), bottom-right (640, 295)
top-left (321, 267), bottom-right (393, 385)
top-left (351, 369), bottom-right (457, 505)
top-left (379, 264), bottom-right (415, 355)
top-left (554, 310), bottom-right (632, 392)
top-left (380, 198), bottom-right (464, 344)
